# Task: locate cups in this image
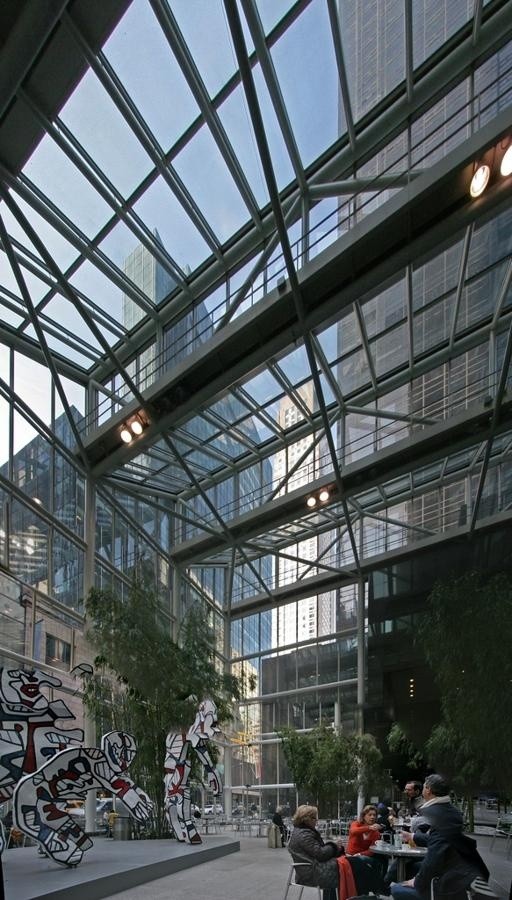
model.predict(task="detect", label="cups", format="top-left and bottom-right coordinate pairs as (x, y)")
top-left (375, 833), bottom-right (416, 850)
top-left (390, 814), bottom-right (426, 832)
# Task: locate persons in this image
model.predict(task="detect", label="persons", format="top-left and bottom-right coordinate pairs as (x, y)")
top-left (108, 809), bottom-right (120, 837)
top-left (103, 810), bottom-right (111, 835)
top-left (272, 806), bottom-right (290, 848)
top-left (287, 773), bottom-right (465, 900)
top-left (194, 807), bottom-right (201, 818)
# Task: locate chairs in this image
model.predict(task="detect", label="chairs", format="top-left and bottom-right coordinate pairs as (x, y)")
top-left (430, 867), bottom-right (477, 900)
top-left (283, 841), bottom-right (339, 900)
top-left (196, 813), bottom-right (352, 842)
